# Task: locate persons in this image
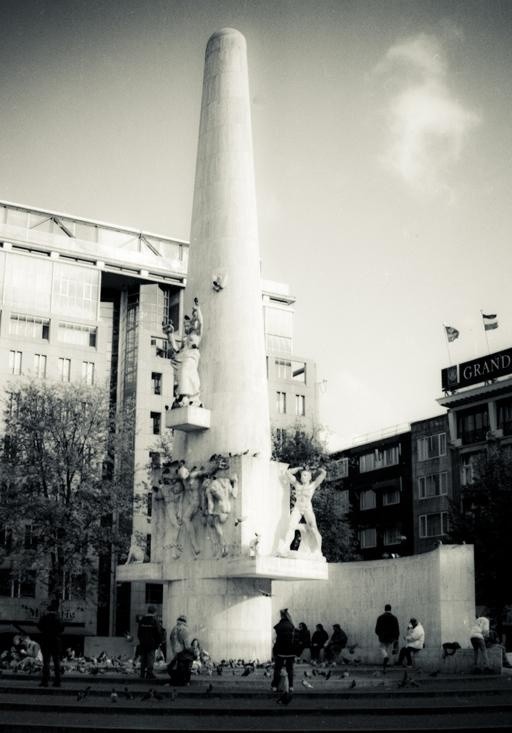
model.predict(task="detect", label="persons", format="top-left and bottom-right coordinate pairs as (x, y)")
top-left (154, 456), bottom-right (238, 560)
top-left (375, 604), bottom-right (399, 667)
top-left (37, 600), bottom-right (64, 688)
top-left (133, 606), bottom-right (167, 680)
top-left (167, 615), bottom-right (209, 686)
top-left (395, 618), bottom-right (425, 668)
top-left (163, 304), bottom-right (202, 407)
top-left (294, 622), bottom-right (347, 666)
top-left (272, 608), bottom-right (296, 692)
top-left (470, 613), bottom-right (494, 674)
top-left (0, 635), bottom-right (43, 671)
top-left (281, 466), bottom-right (326, 559)
top-left (490, 620), bottom-right (512, 668)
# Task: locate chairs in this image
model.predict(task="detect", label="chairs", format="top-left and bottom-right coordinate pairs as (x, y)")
top-left (412, 643), bottom-right (425, 668)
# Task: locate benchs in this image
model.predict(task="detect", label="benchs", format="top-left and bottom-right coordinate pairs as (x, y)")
top-left (300, 647), bottom-right (503, 674)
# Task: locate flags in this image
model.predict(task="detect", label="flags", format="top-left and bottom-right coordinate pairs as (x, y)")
top-left (446, 326), bottom-right (459, 343)
top-left (483, 314), bottom-right (498, 330)
